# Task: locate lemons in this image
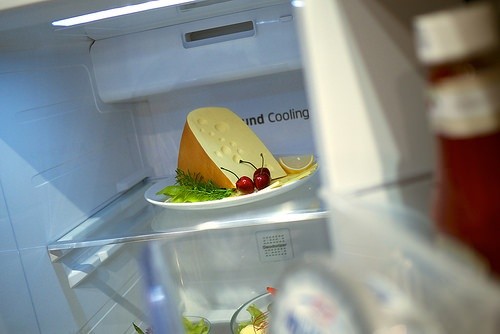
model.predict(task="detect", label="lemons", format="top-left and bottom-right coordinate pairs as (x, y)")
top-left (279, 154), bottom-right (313, 174)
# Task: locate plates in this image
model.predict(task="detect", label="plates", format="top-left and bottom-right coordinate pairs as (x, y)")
top-left (143, 153), bottom-right (318, 209)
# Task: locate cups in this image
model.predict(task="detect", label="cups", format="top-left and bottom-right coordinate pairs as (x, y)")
top-left (230, 292), bottom-right (272, 333)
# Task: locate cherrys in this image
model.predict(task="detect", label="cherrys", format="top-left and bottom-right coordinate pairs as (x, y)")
top-left (239, 158), bottom-right (271, 178)
top-left (255, 152), bottom-right (270, 190)
top-left (220, 166), bottom-right (255, 194)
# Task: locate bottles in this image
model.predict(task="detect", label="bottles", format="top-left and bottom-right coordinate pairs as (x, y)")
top-left (410, 8), bottom-right (500, 276)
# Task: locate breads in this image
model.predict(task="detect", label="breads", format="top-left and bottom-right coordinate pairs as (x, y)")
top-left (177, 106), bottom-right (287, 188)
